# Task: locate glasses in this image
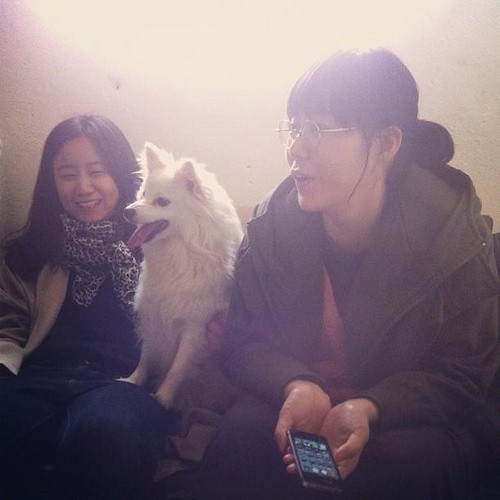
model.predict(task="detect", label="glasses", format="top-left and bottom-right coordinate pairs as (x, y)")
top-left (274, 120), bottom-right (356, 149)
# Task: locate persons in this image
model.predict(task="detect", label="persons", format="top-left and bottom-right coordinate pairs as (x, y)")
top-left (0, 115), bottom-right (178, 500)
top-left (219, 45), bottom-right (500, 500)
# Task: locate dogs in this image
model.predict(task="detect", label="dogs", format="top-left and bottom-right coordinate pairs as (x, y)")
top-left (116, 142), bottom-right (244, 413)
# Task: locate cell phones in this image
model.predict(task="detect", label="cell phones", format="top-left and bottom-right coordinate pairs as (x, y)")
top-left (288, 428), bottom-right (345, 494)
